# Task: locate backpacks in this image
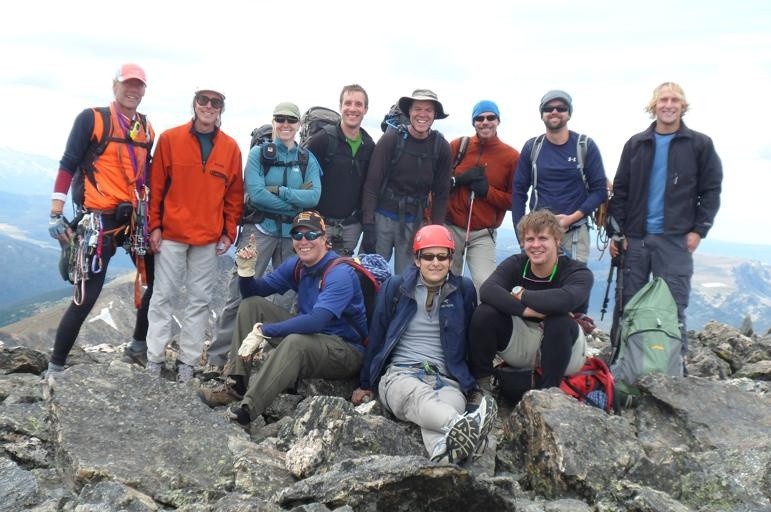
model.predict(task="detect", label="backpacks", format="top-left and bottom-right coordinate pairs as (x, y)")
top-left (606, 277), bottom-right (682, 409)
top-left (299, 106), bottom-right (341, 163)
top-left (241, 124), bottom-right (309, 224)
top-left (381, 105), bottom-right (430, 208)
top-left (294, 252), bottom-right (392, 347)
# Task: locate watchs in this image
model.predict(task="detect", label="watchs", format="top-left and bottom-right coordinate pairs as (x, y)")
top-left (512, 285), bottom-right (525, 298)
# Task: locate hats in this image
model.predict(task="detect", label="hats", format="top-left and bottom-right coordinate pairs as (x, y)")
top-left (472, 100), bottom-right (500, 126)
top-left (193, 84), bottom-right (225, 101)
top-left (398, 89), bottom-right (448, 119)
top-left (116, 64), bottom-right (147, 87)
top-left (274, 102), bottom-right (301, 118)
top-left (539, 91), bottom-right (573, 120)
top-left (289, 210), bottom-right (326, 234)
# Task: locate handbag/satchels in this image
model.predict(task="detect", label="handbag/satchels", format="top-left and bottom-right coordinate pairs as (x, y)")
top-left (495, 357), bottom-right (620, 415)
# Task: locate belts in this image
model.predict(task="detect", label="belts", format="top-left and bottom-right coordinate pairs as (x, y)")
top-left (325, 218), bottom-right (359, 225)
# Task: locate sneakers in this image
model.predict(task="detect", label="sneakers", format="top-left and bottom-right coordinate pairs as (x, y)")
top-left (465, 394), bottom-right (498, 463)
top-left (430, 414), bottom-right (479, 463)
top-left (124, 347), bottom-right (247, 426)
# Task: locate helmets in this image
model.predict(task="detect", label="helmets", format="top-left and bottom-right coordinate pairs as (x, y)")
top-left (413, 225), bottom-right (455, 252)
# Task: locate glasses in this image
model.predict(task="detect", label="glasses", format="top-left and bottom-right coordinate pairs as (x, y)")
top-left (543, 106), bottom-right (568, 112)
top-left (275, 116), bottom-right (298, 123)
top-left (196, 95), bottom-right (223, 110)
top-left (422, 255), bottom-right (448, 261)
top-left (293, 231), bottom-right (323, 240)
top-left (474, 115), bottom-right (497, 122)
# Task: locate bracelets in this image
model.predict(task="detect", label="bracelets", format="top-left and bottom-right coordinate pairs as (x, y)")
top-left (49, 210), bottom-right (64, 218)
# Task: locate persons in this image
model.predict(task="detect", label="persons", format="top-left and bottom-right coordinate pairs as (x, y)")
top-left (197, 209), bottom-right (368, 423)
top-left (603, 81), bottom-right (722, 377)
top-left (349, 223), bottom-right (499, 466)
top-left (142, 84), bottom-right (245, 382)
top-left (43, 63), bottom-right (156, 381)
top-left (421, 99), bottom-right (520, 308)
top-left (299, 85), bottom-right (376, 255)
top-left (199, 100), bottom-right (321, 382)
top-left (511, 90), bottom-right (608, 264)
top-left (358, 86), bottom-right (451, 276)
top-left (464, 207), bottom-right (594, 414)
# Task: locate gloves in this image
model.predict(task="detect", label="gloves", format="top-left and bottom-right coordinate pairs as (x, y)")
top-left (48, 215), bottom-right (73, 243)
top-left (234, 234), bottom-right (256, 277)
top-left (238, 323), bottom-right (272, 357)
